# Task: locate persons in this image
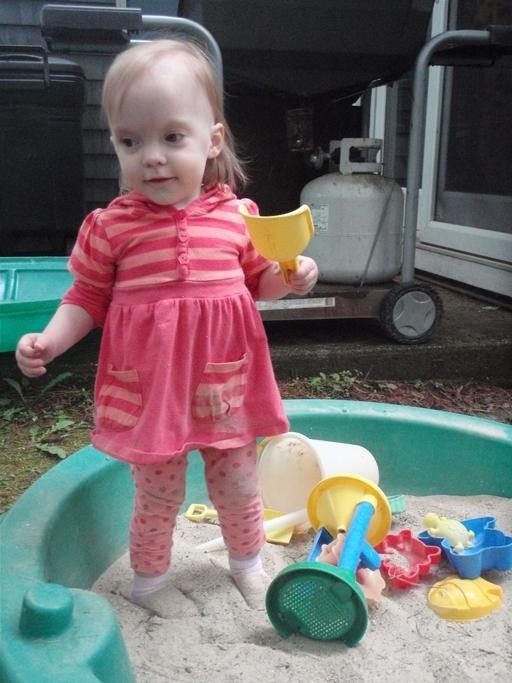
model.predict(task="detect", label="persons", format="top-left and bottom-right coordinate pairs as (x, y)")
top-left (16, 40), bottom-right (318, 619)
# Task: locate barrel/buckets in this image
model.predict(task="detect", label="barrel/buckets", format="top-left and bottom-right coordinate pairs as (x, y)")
top-left (258, 430), bottom-right (380, 531)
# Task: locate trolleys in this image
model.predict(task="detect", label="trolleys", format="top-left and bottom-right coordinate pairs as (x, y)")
top-left (256, 20), bottom-right (510, 346)
